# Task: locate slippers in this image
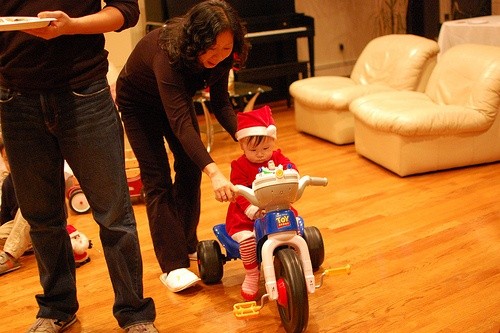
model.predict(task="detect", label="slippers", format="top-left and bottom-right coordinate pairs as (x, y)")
top-left (0, 251), bottom-right (21, 274)
top-left (188, 251), bottom-right (198, 260)
top-left (160, 268), bottom-right (201, 292)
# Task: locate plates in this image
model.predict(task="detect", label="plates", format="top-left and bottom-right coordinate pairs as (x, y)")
top-left (0, 16), bottom-right (59, 31)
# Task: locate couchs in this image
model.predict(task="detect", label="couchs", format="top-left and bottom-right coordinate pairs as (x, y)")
top-left (348, 43), bottom-right (500, 178)
top-left (287, 33), bottom-right (440, 143)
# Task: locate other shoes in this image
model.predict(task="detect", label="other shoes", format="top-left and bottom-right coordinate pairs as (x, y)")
top-left (25, 314), bottom-right (81, 333)
top-left (242, 272), bottom-right (260, 299)
top-left (125, 324), bottom-right (159, 333)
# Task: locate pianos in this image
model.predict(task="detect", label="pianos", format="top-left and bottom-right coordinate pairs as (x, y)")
top-left (143, 0), bottom-right (317, 110)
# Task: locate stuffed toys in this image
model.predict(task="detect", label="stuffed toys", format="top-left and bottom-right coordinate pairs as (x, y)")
top-left (67, 225), bottom-right (93, 267)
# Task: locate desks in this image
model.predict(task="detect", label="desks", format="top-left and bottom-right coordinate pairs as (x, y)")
top-left (437, 15), bottom-right (500, 52)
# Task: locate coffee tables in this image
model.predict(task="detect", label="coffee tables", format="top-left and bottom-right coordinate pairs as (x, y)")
top-left (192, 82), bottom-right (272, 153)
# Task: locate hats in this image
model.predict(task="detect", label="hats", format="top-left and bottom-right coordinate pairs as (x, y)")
top-left (235, 105), bottom-right (277, 140)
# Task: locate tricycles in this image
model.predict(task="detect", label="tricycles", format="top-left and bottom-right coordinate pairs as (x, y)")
top-left (196, 175), bottom-right (329, 333)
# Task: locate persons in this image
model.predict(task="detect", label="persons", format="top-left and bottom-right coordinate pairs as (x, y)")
top-left (0, 140), bottom-right (33, 275)
top-left (225, 107), bottom-right (299, 300)
top-left (116, 0), bottom-right (247, 292)
top-left (0, 0), bottom-right (156, 333)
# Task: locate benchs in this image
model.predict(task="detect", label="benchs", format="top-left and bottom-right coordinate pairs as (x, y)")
top-left (235, 60), bottom-right (310, 110)
